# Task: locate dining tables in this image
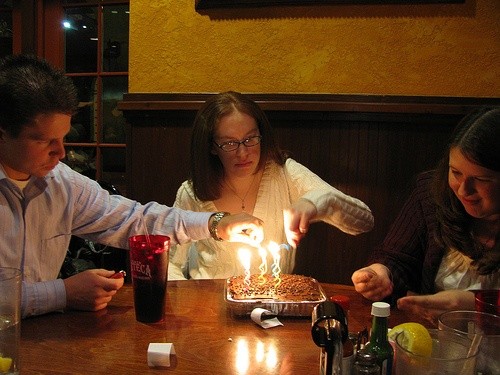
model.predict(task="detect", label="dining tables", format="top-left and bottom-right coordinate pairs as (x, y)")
top-left (0, 279), bottom-right (438, 375)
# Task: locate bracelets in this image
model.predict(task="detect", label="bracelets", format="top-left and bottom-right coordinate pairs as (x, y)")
top-left (209, 211), bottom-right (231, 241)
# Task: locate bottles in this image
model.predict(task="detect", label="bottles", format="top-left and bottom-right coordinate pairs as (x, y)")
top-left (319, 294), bottom-right (396, 375)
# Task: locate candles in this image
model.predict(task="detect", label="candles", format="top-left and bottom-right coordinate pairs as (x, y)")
top-left (238, 240), bottom-right (292, 288)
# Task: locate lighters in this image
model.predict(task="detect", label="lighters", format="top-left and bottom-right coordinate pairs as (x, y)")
top-left (110, 270), bottom-right (127, 279)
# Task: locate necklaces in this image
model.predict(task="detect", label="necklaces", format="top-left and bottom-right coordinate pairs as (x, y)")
top-left (225, 175), bottom-right (256, 210)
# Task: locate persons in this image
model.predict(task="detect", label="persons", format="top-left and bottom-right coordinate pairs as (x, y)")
top-left (351, 108), bottom-right (500, 327)
top-left (163, 91), bottom-right (375, 282)
top-left (0, 54), bottom-right (264, 320)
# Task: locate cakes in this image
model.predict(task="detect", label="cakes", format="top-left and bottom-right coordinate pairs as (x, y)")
top-left (230, 273), bottom-right (321, 301)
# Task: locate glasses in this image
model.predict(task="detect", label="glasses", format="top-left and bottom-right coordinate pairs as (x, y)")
top-left (212, 135), bottom-right (263, 152)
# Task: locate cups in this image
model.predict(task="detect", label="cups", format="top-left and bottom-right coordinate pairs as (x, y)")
top-left (474, 289), bottom-right (500, 327)
top-left (437, 310), bottom-right (500, 375)
top-left (127, 234), bottom-right (171, 324)
top-left (0, 266), bottom-right (23, 375)
top-left (396, 328), bottom-right (481, 375)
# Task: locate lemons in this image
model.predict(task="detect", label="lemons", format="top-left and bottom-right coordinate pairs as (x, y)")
top-left (387, 322), bottom-right (433, 363)
top-left (0, 357), bottom-right (13, 372)
